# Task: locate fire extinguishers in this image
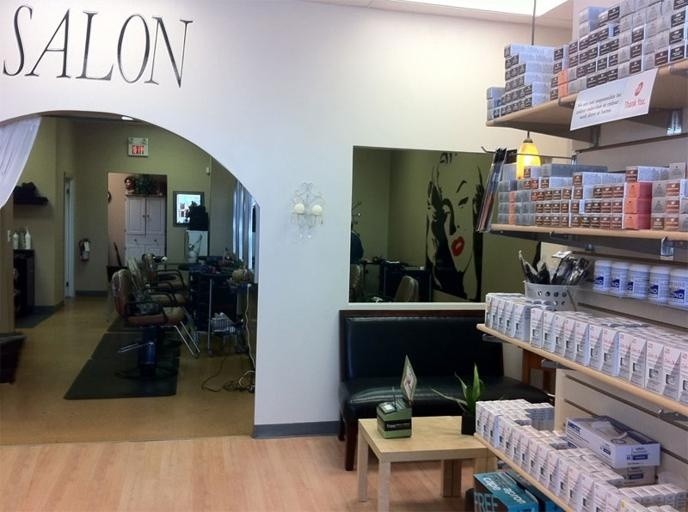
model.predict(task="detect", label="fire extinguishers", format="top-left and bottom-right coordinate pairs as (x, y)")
top-left (79, 238), bottom-right (91, 262)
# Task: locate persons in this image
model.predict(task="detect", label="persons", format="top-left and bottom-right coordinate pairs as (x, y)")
top-left (423, 149), bottom-right (483, 301)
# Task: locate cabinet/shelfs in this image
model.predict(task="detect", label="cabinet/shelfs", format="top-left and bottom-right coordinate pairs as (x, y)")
top-left (124, 194), bottom-right (166, 264)
top-left (474, 60), bottom-right (688, 512)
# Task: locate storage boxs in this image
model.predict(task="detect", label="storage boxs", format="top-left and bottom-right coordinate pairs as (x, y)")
top-left (473, 472), bottom-right (540, 512)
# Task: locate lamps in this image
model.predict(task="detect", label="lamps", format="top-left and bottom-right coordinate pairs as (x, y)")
top-left (514, 0), bottom-right (542, 178)
top-left (290, 181), bottom-right (326, 238)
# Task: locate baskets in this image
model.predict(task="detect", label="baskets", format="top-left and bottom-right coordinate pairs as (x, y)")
top-left (522, 279), bottom-right (580, 312)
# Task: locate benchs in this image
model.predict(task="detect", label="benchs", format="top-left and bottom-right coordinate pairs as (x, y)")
top-left (338, 310), bottom-right (550, 470)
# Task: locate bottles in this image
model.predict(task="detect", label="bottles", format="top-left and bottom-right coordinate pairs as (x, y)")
top-left (625, 263), bottom-right (651, 300)
top-left (648, 265), bottom-right (671, 304)
top-left (608, 262), bottom-right (630, 296)
top-left (592, 259), bottom-right (612, 293)
top-left (668, 268), bottom-right (688, 308)
top-left (12, 227), bottom-right (32, 250)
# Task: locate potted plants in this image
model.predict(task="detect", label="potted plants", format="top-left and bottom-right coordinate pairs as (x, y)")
top-left (132, 174), bottom-right (157, 197)
top-left (432, 362), bottom-right (487, 434)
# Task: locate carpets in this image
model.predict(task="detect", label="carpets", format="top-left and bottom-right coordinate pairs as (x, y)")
top-left (63, 317), bottom-right (181, 399)
top-left (15, 309), bottom-right (54, 328)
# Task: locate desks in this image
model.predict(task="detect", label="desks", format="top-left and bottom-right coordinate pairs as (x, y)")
top-left (358, 415), bottom-right (497, 512)
top-left (14, 249), bottom-right (35, 316)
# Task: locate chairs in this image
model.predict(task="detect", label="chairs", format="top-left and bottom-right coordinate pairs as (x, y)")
top-left (113, 252), bottom-right (201, 381)
top-left (394, 276), bottom-right (419, 302)
top-left (349, 264), bottom-right (365, 302)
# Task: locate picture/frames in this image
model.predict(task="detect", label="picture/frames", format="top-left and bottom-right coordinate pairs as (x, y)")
top-left (173, 189), bottom-right (204, 228)
